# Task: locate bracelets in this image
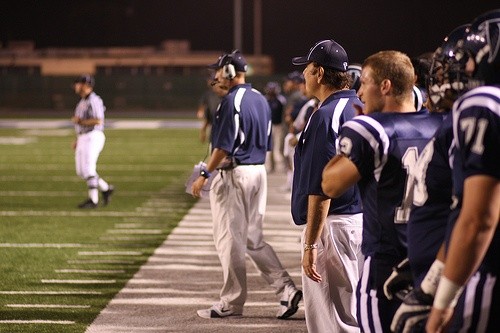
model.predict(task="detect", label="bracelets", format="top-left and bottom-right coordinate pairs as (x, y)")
top-left (304, 244), bottom-right (318, 250)
top-left (421, 259), bottom-right (445, 295)
top-left (434, 275), bottom-right (464, 310)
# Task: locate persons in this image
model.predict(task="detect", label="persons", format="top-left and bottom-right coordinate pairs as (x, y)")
top-left (73, 74), bottom-right (114, 209)
top-left (322, 51), bottom-right (444, 333)
top-left (192, 49), bottom-right (302, 318)
top-left (292, 39), bottom-right (363, 333)
top-left (384, 19), bottom-right (500, 333)
top-left (263, 72), bottom-right (320, 173)
top-left (200, 73), bottom-right (227, 153)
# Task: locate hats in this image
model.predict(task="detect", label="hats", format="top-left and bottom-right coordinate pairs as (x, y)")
top-left (291, 39), bottom-right (349, 72)
top-left (206, 53), bottom-right (248, 72)
top-left (73, 73), bottom-right (94, 84)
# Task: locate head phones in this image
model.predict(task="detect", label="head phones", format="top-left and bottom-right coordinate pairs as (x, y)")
top-left (223, 54), bottom-right (236, 80)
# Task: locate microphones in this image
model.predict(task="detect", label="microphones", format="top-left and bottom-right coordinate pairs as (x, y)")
top-left (211, 81), bottom-right (219, 86)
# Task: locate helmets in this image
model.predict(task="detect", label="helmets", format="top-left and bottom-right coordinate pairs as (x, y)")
top-left (413, 53), bottom-right (435, 89)
top-left (426, 24), bottom-right (471, 110)
top-left (452, 9), bottom-right (500, 97)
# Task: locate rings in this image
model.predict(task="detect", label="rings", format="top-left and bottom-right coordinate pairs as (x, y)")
top-left (307, 273), bottom-right (309, 275)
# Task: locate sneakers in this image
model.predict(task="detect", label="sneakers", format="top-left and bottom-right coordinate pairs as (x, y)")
top-left (78, 198), bottom-right (99, 209)
top-left (276, 284), bottom-right (303, 319)
top-left (101, 184), bottom-right (114, 207)
top-left (196, 303), bottom-right (242, 318)
